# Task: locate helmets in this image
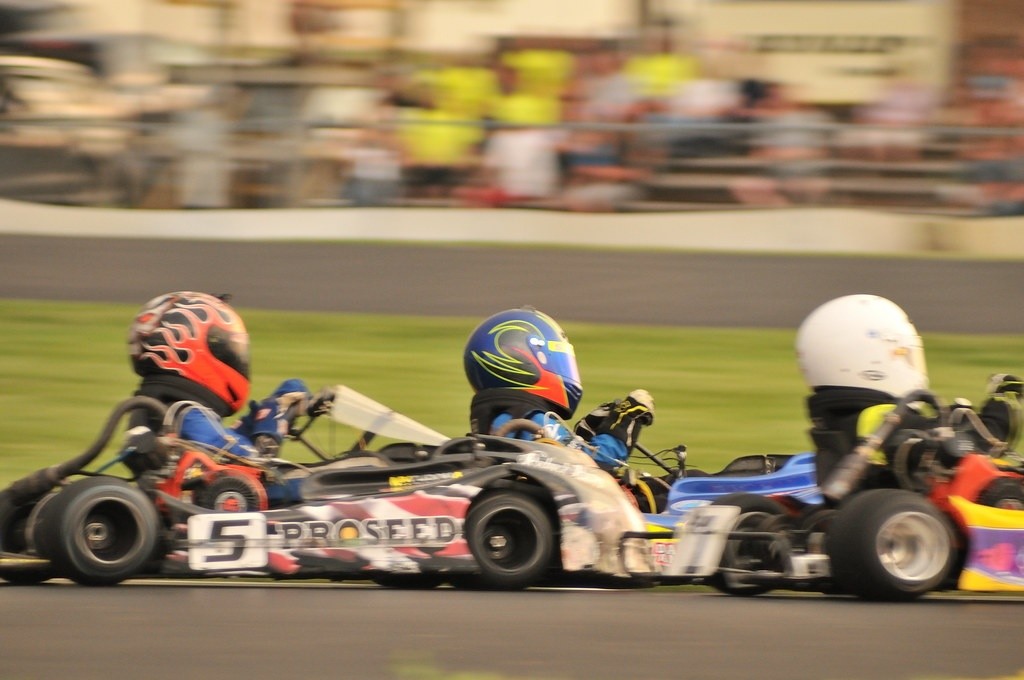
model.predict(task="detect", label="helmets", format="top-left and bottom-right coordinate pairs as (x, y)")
top-left (796, 295), bottom-right (926, 403)
top-left (128, 292), bottom-right (252, 412)
top-left (463, 308), bottom-right (584, 416)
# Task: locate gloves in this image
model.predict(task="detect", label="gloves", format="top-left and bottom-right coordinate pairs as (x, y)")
top-left (597, 388), bottom-right (654, 448)
top-left (571, 399), bottom-right (619, 441)
top-left (253, 378), bottom-right (312, 443)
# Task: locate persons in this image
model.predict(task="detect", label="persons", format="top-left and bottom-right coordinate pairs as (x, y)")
top-left (790, 289), bottom-right (970, 491)
top-left (462, 305), bottom-right (656, 476)
top-left (128, 289), bottom-right (309, 466)
top-left (339, 4), bottom-right (1023, 202)
